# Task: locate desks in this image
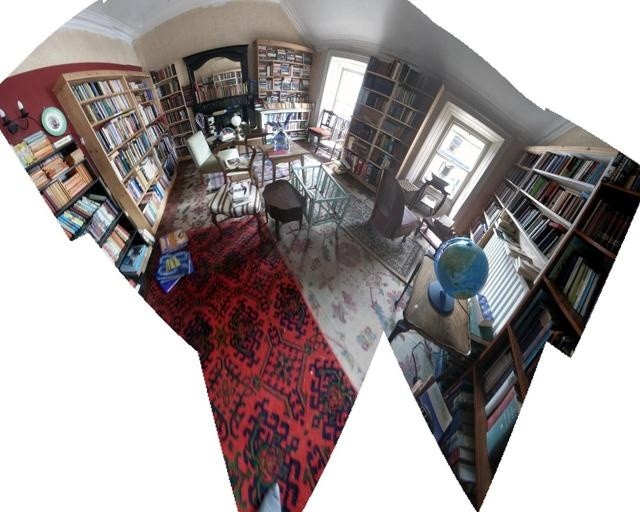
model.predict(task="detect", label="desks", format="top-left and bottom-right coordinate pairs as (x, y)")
top-left (386, 254), bottom-right (472, 387)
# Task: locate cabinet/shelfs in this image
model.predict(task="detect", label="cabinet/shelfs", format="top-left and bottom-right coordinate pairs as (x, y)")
top-left (291, 164), bottom-right (352, 240)
top-left (0, 38), bottom-right (317, 300)
top-left (410, 141), bottom-right (639, 512)
top-left (335, 55), bottom-right (444, 195)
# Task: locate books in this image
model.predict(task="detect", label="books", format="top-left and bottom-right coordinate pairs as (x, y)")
top-left (12, 65), bottom-right (195, 296)
top-left (194, 46), bottom-right (425, 209)
top-left (412, 152), bottom-right (640, 512)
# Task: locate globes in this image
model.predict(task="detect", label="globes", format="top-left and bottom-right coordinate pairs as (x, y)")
top-left (428, 237), bottom-right (489, 315)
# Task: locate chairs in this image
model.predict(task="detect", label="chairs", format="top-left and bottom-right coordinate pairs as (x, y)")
top-left (368, 167), bottom-right (464, 259)
top-left (306, 109), bottom-right (352, 168)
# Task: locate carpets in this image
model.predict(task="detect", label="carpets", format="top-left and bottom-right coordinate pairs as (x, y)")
top-left (143, 215), bottom-right (357, 512)
